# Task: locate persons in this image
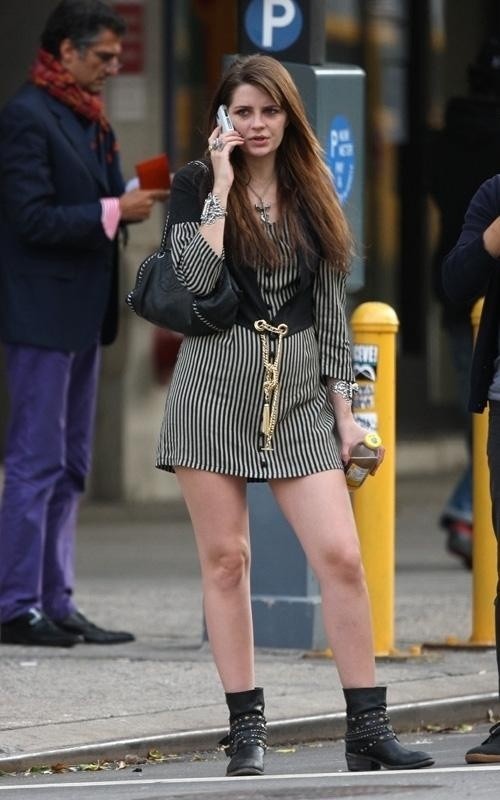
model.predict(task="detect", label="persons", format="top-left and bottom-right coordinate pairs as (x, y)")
top-left (440, 176), bottom-right (500, 764)
top-left (1, 0), bottom-right (175, 649)
top-left (427, 50), bottom-right (500, 563)
top-left (135, 53), bottom-right (435, 778)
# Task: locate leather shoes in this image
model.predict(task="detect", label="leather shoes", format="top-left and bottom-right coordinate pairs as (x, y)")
top-left (1, 607), bottom-right (79, 649)
top-left (44, 610), bottom-right (135, 645)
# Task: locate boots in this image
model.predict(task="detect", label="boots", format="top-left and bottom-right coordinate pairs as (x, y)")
top-left (224, 686), bottom-right (267, 777)
top-left (342, 686), bottom-right (436, 772)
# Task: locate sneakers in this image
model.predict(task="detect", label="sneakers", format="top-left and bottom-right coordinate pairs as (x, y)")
top-left (465, 722), bottom-right (499, 765)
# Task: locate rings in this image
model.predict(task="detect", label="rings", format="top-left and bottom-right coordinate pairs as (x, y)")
top-left (206, 138), bottom-right (224, 154)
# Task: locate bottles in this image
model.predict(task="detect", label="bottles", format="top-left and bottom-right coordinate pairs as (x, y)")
top-left (348, 427), bottom-right (382, 496)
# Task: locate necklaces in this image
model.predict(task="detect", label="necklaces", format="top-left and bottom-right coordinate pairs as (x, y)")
top-left (240, 185), bottom-right (277, 224)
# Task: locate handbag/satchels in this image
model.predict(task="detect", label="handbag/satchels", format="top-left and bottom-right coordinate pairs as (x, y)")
top-left (125, 158), bottom-right (242, 337)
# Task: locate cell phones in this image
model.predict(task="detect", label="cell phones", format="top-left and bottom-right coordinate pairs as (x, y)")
top-left (216, 104), bottom-right (234, 135)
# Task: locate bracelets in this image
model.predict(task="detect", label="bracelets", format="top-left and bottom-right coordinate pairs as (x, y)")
top-left (326, 379), bottom-right (361, 403)
top-left (200, 193), bottom-right (229, 226)
